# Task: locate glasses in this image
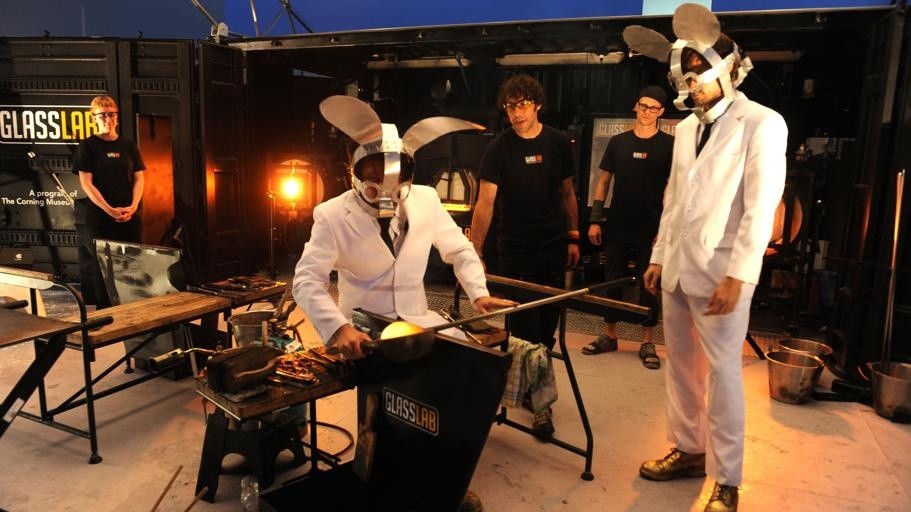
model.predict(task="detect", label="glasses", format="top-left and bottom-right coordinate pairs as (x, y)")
top-left (93, 112), bottom-right (117, 117)
top-left (502, 99), bottom-right (531, 110)
top-left (638, 102), bottom-right (663, 113)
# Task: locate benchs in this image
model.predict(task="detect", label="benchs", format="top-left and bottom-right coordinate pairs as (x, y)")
top-left (191, 311), bottom-right (508, 505)
top-left (29, 273), bottom-right (291, 465)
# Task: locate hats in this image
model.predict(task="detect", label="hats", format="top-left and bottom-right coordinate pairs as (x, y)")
top-left (641, 86), bottom-right (666, 106)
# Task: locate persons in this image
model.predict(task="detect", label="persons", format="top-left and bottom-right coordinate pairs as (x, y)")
top-left (640, 31), bottom-right (791, 512)
top-left (72, 95), bottom-right (146, 311)
top-left (292, 132), bottom-right (520, 511)
top-left (470, 74), bottom-right (579, 441)
top-left (580, 87), bottom-right (678, 368)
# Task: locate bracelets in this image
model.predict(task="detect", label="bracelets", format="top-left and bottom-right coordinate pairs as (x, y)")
top-left (589, 199), bottom-right (605, 226)
top-left (567, 230), bottom-right (579, 246)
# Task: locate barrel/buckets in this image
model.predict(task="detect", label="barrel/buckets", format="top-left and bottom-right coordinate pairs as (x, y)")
top-left (869, 362), bottom-right (911, 422)
top-left (776, 337), bottom-right (834, 365)
top-left (229, 311), bottom-right (290, 346)
top-left (764, 350), bottom-right (825, 405)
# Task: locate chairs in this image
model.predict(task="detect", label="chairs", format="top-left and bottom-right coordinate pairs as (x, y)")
top-left (0, 270), bottom-right (101, 465)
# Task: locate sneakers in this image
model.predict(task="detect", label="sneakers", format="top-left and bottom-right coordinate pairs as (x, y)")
top-left (581, 335), bottom-right (617, 354)
top-left (533, 407), bottom-right (555, 441)
top-left (640, 343), bottom-right (660, 369)
top-left (640, 448), bottom-right (706, 480)
top-left (700, 480), bottom-right (738, 511)
